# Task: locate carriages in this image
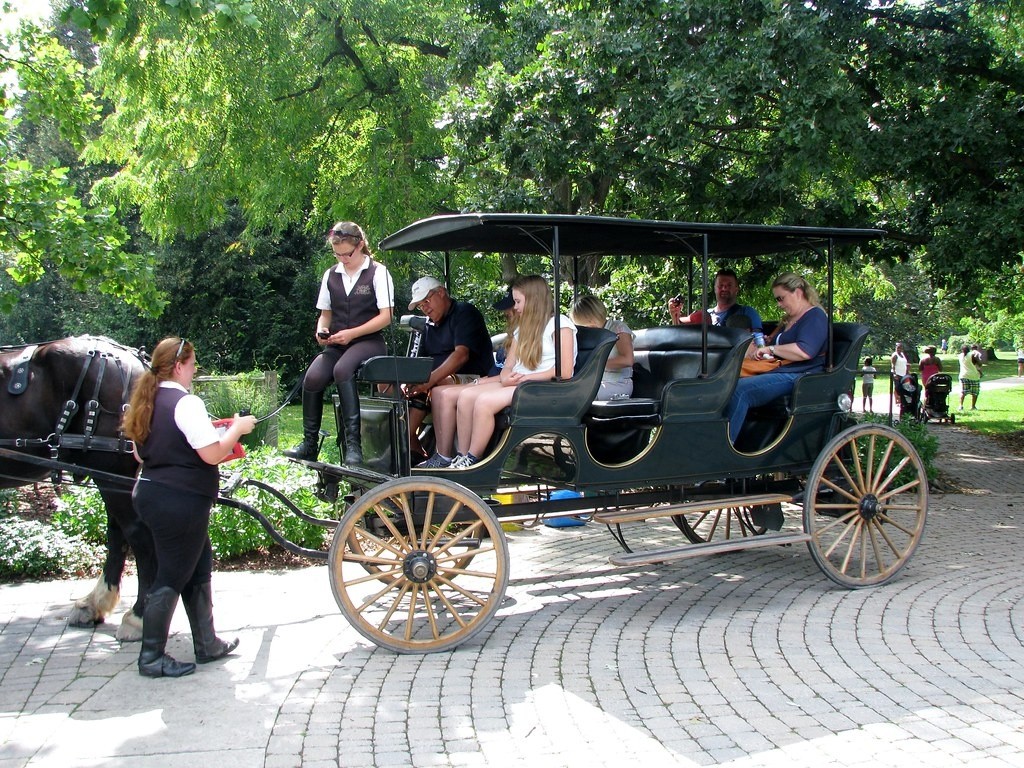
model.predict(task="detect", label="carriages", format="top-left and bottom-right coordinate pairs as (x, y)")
top-left (1, 213), bottom-right (931, 654)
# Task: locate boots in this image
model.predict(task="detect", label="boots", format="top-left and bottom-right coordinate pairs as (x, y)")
top-left (183, 579), bottom-right (240, 664)
top-left (281, 386), bottom-right (324, 462)
top-left (335, 375), bottom-right (364, 466)
top-left (138, 586), bottom-right (196, 678)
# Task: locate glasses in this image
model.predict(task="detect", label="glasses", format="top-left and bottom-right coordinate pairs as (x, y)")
top-left (175, 337), bottom-right (188, 365)
top-left (333, 240), bottom-right (364, 258)
top-left (773, 292), bottom-right (790, 302)
top-left (416, 291), bottom-right (439, 310)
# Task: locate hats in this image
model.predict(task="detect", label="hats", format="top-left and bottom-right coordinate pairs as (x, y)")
top-left (492, 285), bottom-right (516, 311)
top-left (407, 276), bottom-right (444, 311)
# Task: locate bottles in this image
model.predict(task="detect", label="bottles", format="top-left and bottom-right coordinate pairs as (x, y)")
top-left (752, 330), bottom-right (765, 348)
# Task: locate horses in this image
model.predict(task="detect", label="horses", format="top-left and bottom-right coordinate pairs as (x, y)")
top-left (0, 335), bottom-right (159, 643)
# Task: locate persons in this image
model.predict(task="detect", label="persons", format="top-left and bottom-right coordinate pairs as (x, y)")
top-left (415, 275), bottom-right (577, 469)
top-left (1017, 348), bottom-right (1024, 377)
top-left (941, 339), bottom-right (948, 354)
top-left (376, 277), bottom-right (494, 460)
top-left (861, 343), bottom-right (943, 414)
top-left (132, 337), bottom-right (258, 677)
top-left (958, 345), bottom-right (983, 410)
top-left (283, 222), bottom-right (394, 467)
top-left (668, 268), bottom-right (829, 446)
top-left (570, 296), bottom-right (635, 402)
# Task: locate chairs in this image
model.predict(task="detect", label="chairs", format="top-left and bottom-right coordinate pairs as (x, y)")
top-left (355, 313), bottom-right (434, 383)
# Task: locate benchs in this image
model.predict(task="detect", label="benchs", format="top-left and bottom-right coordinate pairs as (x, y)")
top-left (749, 320), bottom-right (873, 415)
top-left (490, 325), bottom-right (620, 428)
top-left (631, 325), bottom-right (754, 424)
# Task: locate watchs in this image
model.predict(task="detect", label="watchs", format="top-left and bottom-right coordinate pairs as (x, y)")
top-left (769, 345), bottom-right (774, 356)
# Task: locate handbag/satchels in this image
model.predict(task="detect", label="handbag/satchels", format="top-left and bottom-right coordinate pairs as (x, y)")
top-left (739, 336), bottom-right (791, 377)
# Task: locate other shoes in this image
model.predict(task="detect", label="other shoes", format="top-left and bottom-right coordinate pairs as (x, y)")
top-left (957, 406), bottom-right (963, 410)
top-left (971, 407), bottom-right (977, 410)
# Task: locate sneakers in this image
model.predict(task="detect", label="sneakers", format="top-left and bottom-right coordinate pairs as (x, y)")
top-left (442, 452), bottom-right (483, 470)
top-left (414, 450), bottom-right (451, 469)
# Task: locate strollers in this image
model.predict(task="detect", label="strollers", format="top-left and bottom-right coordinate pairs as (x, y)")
top-left (894, 373), bottom-right (925, 427)
top-left (923, 372), bottom-right (955, 425)
